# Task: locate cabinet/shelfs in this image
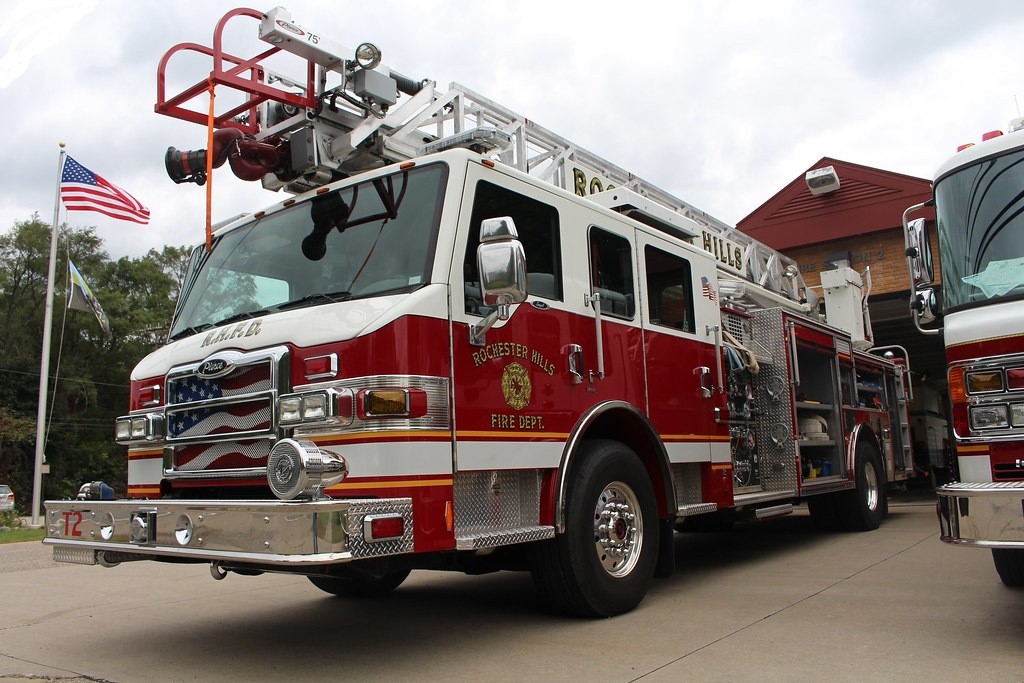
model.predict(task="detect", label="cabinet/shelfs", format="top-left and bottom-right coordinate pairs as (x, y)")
top-left (790, 340), bottom-right (847, 485)
top-left (855, 358), bottom-right (885, 409)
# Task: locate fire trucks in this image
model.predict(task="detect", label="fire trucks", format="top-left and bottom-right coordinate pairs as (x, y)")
top-left (42, 5), bottom-right (916, 617)
top-left (901, 119), bottom-right (1024, 589)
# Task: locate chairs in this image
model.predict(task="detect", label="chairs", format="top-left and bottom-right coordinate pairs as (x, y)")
top-left (526, 273), bottom-right (555, 298)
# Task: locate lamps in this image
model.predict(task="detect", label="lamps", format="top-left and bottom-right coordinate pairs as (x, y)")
top-left (806, 165), bottom-right (841, 196)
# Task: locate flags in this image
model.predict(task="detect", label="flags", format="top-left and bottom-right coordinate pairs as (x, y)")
top-left (61, 155), bottom-right (150, 226)
top-left (68, 261), bottom-right (110, 333)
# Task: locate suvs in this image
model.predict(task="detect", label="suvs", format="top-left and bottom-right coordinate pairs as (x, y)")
top-left (0, 485), bottom-right (14, 513)
